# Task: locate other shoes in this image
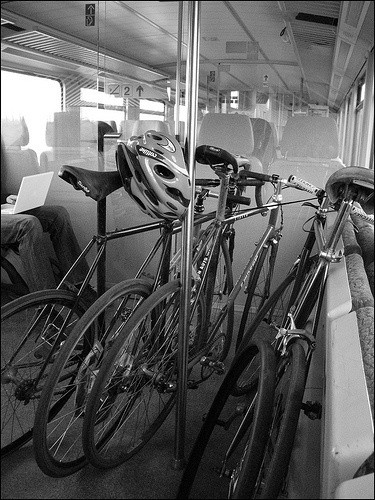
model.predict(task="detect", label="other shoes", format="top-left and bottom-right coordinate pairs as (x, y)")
top-left (35, 308), bottom-right (64, 339)
top-left (65, 283), bottom-right (100, 310)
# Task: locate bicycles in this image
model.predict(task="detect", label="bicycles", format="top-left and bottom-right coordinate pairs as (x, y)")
top-left (0, 144), bottom-right (375, 500)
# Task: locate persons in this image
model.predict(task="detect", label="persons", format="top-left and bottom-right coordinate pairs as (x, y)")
top-left (1, 193), bottom-right (115, 342)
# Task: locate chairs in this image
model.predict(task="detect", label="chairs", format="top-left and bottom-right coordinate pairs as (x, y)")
top-left (39, 113), bottom-right (348, 238)
top-left (0, 113), bottom-right (39, 195)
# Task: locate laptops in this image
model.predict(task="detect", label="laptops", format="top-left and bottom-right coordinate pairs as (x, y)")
top-left (1, 171), bottom-right (54, 214)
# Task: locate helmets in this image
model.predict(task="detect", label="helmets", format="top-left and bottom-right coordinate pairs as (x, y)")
top-left (116, 130), bottom-right (193, 221)
